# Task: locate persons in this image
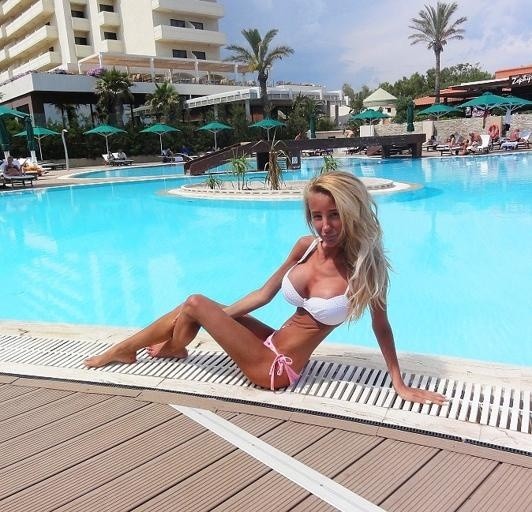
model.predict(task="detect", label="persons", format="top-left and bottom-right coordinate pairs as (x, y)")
top-left (83, 170), bottom-right (449, 409)
top-left (164, 147), bottom-right (176, 164)
top-left (180, 144), bottom-right (193, 161)
top-left (294, 130), bottom-right (305, 158)
top-left (117, 149), bottom-right (128, 166)
top-left (106, 151), bottom-right (119, 161)
top-left (426, 127), bottom-right (521, 157)
top-left (4, 156), bottom-right (24, 177)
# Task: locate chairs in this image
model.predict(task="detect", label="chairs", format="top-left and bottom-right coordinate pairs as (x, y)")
top-left (157, 149), bottom-right (201, 162)
top-left (422, 128), bottom-right (532, 156)
top-left (102, 151), bottom-right (134, 167)
top-left (301, 144), bottom-right (382, 156)
top-left (0, 158), bottom-right (65, 191)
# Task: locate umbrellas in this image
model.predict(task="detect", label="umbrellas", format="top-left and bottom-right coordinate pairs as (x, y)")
top-left (138, 122), bottom-right (182, 155)
top-left (195, 121), bottom-right (235, 148)
top-left (498, 94), bottom-right (531, 114)
top-left (13, 127), bottom-right (62, 162)
top-left (351, 110), bottom-right (391, 135)
top-left (248, 120), bottom-right (288, 141)
top-left (417, 103), bottom-right (462, 120)
top-left (455, 91), bottom-right (520, 115)
top-left (83, 123), bottom-right (129, 154)
top-left (0, 105), bottom-right (31, 120)
